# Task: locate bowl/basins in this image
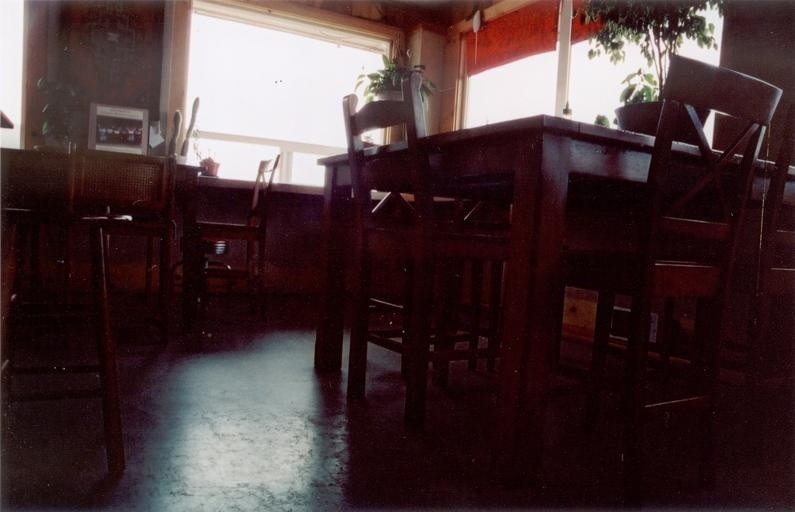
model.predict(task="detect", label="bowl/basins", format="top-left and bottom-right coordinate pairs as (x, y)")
top-left (198, 161), bottom-right (219, 176)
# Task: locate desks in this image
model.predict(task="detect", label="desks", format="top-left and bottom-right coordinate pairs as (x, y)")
top-left (311, 113), bottom-right (795, 489)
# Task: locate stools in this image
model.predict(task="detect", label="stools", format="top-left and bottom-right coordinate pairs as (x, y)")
top-left (6, 206), bottom-right (135, 476)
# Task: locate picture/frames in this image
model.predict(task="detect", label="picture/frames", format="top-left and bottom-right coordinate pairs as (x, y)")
top-left (86, 100), bottom-right (151, 157)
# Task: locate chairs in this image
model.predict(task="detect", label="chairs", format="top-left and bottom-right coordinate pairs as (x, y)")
top-left (1, 152), bottom-right (282, 347)
top-left (344, 50), bottom-right (794, 511)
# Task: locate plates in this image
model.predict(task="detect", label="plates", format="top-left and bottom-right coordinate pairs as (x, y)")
top-left (198, 176), bottom-right (219, 179)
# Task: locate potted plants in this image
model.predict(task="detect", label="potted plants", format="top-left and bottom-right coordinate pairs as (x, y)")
top-left (33, 73), bottom-right (82, 153)
top-left (571, 0), bottom-right (734, 149)
